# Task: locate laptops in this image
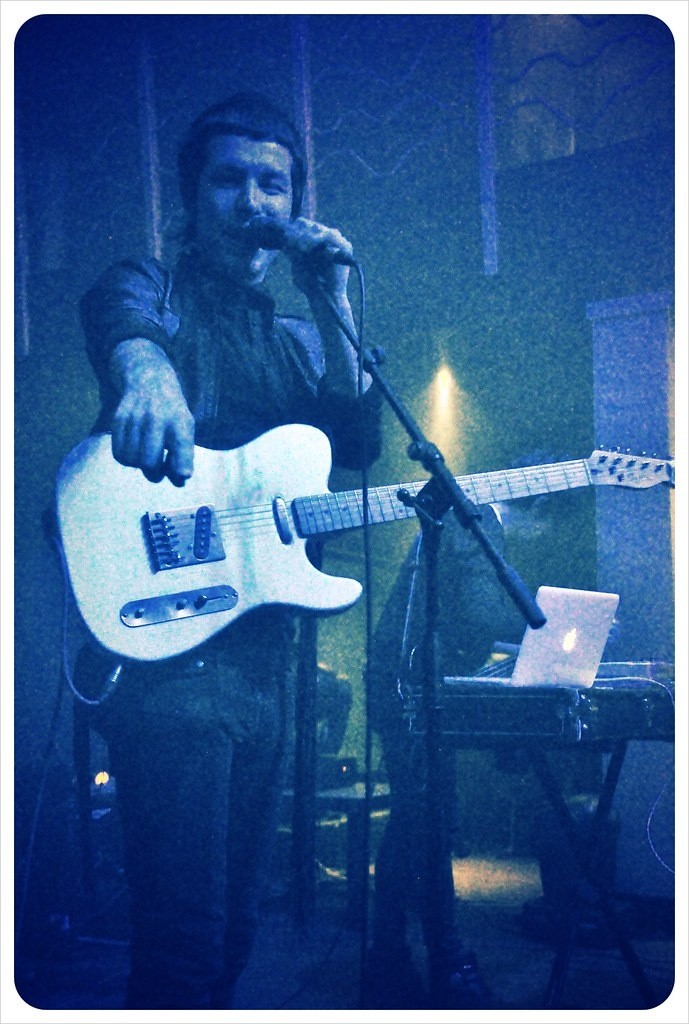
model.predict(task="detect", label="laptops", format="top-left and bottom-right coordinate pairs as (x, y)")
top-left (444, 583), bottom-right (621, 688)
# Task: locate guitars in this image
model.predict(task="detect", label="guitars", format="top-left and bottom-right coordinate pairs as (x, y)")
top-left (56, 424), bottom-right (676, 660)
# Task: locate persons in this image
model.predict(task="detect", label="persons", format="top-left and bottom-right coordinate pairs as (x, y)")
top-left (365, 489), bottom-right (530, 985)
top-left (76, 93), bottom-right (383, 1010)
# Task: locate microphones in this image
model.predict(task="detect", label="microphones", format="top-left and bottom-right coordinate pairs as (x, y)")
top-left (241, 215), bottom-right (358, 269)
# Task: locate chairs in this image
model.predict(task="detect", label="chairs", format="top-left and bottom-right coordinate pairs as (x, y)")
top-left (269, 665), bottom-right (390, 936)
